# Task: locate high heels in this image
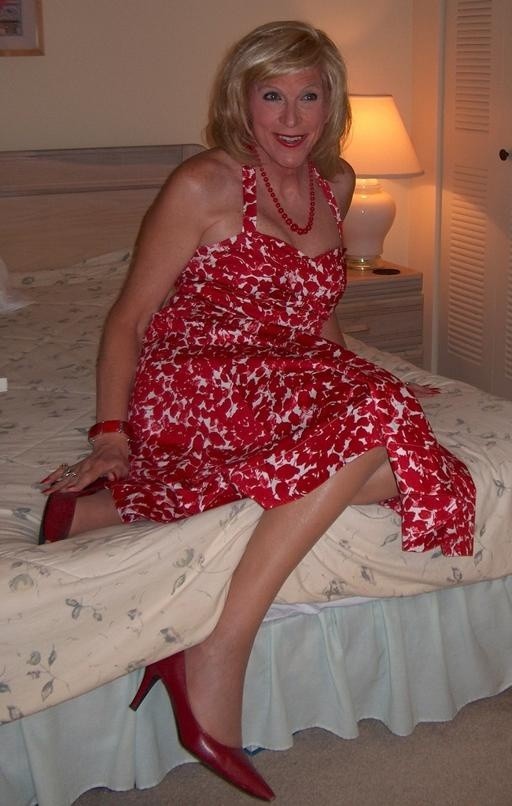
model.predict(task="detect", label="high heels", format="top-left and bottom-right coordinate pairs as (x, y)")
top-left (38, 477), bottom-right (108, 544)
top-left (129, 651), bottom-right (278, 801)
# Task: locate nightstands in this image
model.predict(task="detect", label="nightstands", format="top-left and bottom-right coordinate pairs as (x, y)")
top-left (334, 260), bottom-right (425, 371)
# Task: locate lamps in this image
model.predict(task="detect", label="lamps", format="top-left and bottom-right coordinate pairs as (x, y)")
top-left (339, 93), bottom-right (425, 271)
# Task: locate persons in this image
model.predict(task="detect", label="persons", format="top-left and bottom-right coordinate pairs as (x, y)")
top-left (37, 16), bottom-right (473, 802)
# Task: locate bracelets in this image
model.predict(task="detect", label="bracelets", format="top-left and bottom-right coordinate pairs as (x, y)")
top-left (86, 419), bottom-right (132, 447)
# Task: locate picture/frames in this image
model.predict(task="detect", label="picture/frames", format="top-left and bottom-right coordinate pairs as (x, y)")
top-left (0, 0), bottom-right (46, 56)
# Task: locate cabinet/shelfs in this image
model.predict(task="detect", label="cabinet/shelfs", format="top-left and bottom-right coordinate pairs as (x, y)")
top-left (438, 0), bottom-right (512, 401)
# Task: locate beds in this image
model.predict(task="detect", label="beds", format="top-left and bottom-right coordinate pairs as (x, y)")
top-left (0, 141), bottom-right (511, 803)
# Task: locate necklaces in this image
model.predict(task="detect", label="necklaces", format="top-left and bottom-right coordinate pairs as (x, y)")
top-left (249, 135), bottom-right (317, 235)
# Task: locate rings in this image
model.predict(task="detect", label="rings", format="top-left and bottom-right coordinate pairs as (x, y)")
top-left (59, 463), bottom-right (69, 469)
top-left (63, 467), bottom-right (74, 478)
top-left (66, 473), bottom-right (80, 481)
top-left (403, 380), bottom-right (410, 386)
